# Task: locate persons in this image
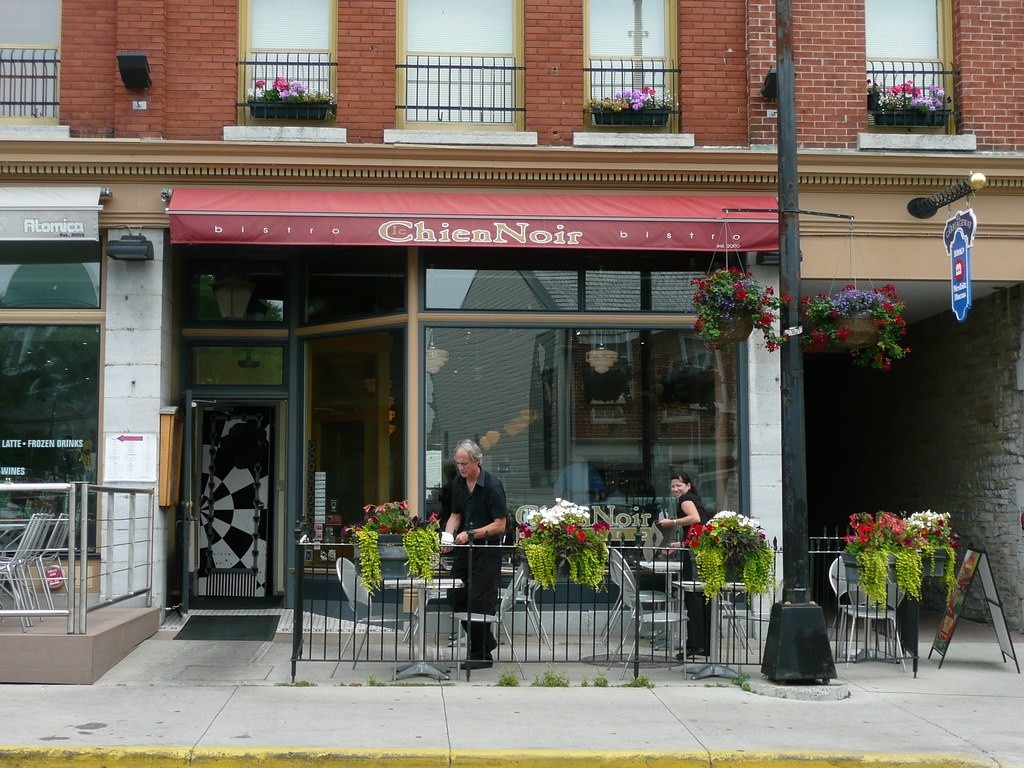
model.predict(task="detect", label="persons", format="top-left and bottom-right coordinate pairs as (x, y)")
top-left (659, 471), bottom-right (713, 659)
top-left (435, 439), bottom-right (508, 669)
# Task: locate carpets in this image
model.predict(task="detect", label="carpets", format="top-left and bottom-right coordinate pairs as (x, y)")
top-left (173, 613), bottom-right (281, 642)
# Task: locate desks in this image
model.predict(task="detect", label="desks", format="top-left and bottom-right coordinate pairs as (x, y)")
top-left (672, 581), bottom-right (747, 679)
top-left (386, 579), bottom-right (464, 680)
top-left (642, 561), bottom-right (685, 649)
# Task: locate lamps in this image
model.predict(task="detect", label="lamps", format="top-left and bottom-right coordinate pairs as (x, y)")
top-left (758, 249), bottom-right (804, 265)
top-left (115, 54), bottom-right (151, 91)
top-left (480, 409), bottom-right (536, 451)
top-left (762, 67), bottom-right (797, 99)
top-left (425, 329), bottom-right (448, 373)
top-left (586, 329), bottom-right (617, 374)
top-left (106, 235), bottom-right (155, 260)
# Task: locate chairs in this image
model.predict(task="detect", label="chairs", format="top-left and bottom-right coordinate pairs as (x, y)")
top-left (0, 513), bottom-right (70, 633)
top-left (830, 556), bottom-right (907, 673)
top-left (602, 549), bottom-right (689, 680)
top-left (451, 558), bottom-right (553, 679)
top-left (331, 558), bottom-right (417, 682)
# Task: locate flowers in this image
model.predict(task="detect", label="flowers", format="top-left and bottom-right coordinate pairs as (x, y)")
top-left (695, 266), bottom-right (791, 352)
top-left (517, 497), bottom-right (610, 547)
top-left (249, 77), bottom-right (332, 101)
top-left (867, 79), bottom-right (951, 108)
top-left (587, 86), bottom-right (676, 108)
top-left (847, 510), bottom-right (959, 551)
top-left (358, 502), bottom-right (417, 534)
top-left (686, 512), bottom-right (766, 546)
top-left (802, 283), bottom-right (910, 373)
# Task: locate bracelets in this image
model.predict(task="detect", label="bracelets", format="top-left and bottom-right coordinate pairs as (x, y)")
top-left (673, 519), bottom-right (677, 526)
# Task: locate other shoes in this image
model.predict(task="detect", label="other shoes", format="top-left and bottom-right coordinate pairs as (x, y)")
top-left (461, 658), bottom-right (492, 669)
top-left (676, 648), bottom-right (704, 660)
top-left (490, 640), bottom-right (497, 651)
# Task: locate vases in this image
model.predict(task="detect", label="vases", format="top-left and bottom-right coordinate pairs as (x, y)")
top-left (249, 103), bottom-right (330, 120)
top-left (715, 320), bottom-right (753, 341)
top-left (832, 316), bottom-right (877, 346)
top-left (841, 551), bottom-right (947, 584)
top-left (725, 563), bottom-right (745, 582)
top-left (378, 535), bottom-right (404, 546)
top-left (591, 110), bottom-right (668, 125)
top-left (874, 110), bottom-right (949, 126)
top-left (555, 552), bottom-right (571, 576)
